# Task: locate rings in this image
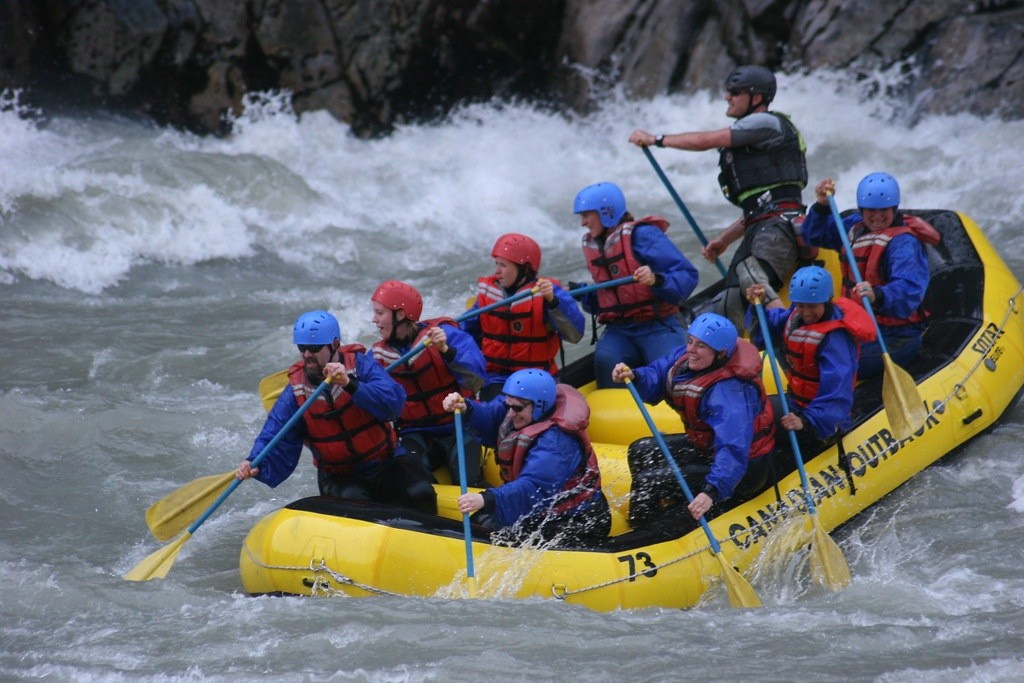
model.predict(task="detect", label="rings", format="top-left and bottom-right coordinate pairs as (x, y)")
top-left (702, 509), bottom-right (705, 512)
top-left (862, 286), bottom-right (866, 292)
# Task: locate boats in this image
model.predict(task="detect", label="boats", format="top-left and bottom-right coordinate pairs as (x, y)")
top-left (239, 208), bottom-right (1023, 615)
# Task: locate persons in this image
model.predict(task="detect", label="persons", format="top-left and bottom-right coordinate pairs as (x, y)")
top-left (234, 308), bottom-right (438, 520)
top-left (442, 369), bottom-right (612, 546)
top-left (613, 311), bottom-right (776, 528)
top-left (454, 233), bottom-right (586, 401)
top-left (799, 173), bottom-right (932, 383)
top-left (629, 64), bottom-right (810, 332)
top-left (364, 280), bottom-right (489, 488)
top-left (562, 182), bottom-right (700, 388)
top-left (745, 266), bottom-right (878, 468)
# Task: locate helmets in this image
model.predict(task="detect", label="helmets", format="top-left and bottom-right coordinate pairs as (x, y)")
top-left (725, 65), bottom-right (777, 105)
top-left (292, 310), bottom-right (341, 346)
top-left (573, 182), bottom-right (628, 228)
top-left (491, 233), bottom-right (541, 274)
top-left (504, 368), bottom-right (557, 422)
top-left (857, 172), bottom-right (900, 208)
top-left (687, 313), bottom-right (738, 357)
top-left (371, 282), bottom-right (423, 322)
top-left (789, 268), bottom-right (833, 304)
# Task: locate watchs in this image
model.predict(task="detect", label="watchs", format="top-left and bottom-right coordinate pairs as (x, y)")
top-left (655, 134), bottom-right (665, 148)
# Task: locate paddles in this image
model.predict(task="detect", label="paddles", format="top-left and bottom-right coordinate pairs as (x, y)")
top-left (466, 275), bottom-right (656, 310)
top-left (258, 278), bottom-right (543, 414)
top-left (746, 289), bottom-right (851, 593)
top-left (454, 398), bottom-right (477, 598)
top-left (822, 181), bottom-right (928, 441)
top-left (623, 366), bottom-right (762, 609)
top-left (146, 468), bottom-right (239, 542)
top-left (123, 374), bottom-right (341, 581)
top-left (639, 141), bottom-right (728, 278)
top-left (384, 336), bottom-right (443, 373)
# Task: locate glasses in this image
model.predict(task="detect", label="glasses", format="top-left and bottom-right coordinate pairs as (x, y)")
top-left (727, 88), bottom-right (750, 96)
top-left (297, 344), bottom-right (329, 354)
top-left (502, 399), bottom-right (534, 412)
